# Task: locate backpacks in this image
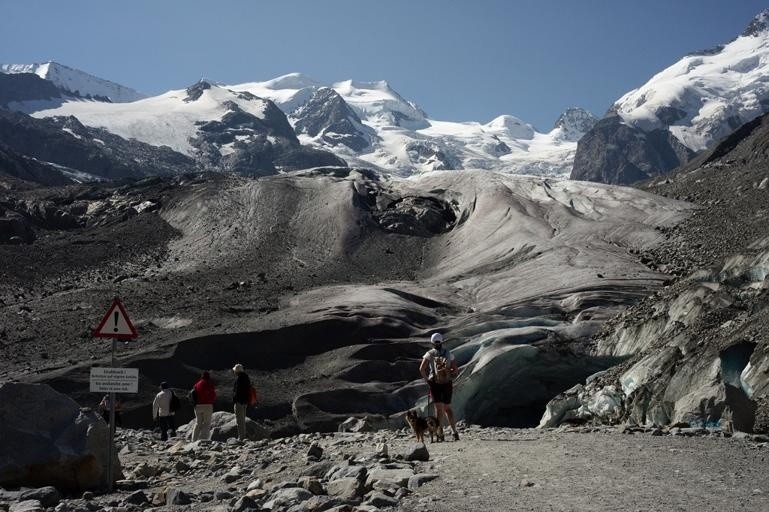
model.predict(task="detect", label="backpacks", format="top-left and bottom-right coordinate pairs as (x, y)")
top-left (427, 349), bottom-right (450, 385)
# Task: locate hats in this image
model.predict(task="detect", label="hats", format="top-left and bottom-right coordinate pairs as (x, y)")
top-left (233, 364), bottom-right (244, 373)
top-left (431, 333), bottom-right (442, 344)
top-left (201, 372), bottom-right (210, 380)
top-left (160, 382), bottom-right (168, 389)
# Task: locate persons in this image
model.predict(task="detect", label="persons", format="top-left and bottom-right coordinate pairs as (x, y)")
top-left (190, 371), bottom-right (217, 443)
top-left (152, 381), bottom-right (177, 441)
top-left (232, 363), bottom-right (251, 441)
top-left (419, 332), bottom-right (460, 442)
top-left (99, 392), bottom-right (124, 433)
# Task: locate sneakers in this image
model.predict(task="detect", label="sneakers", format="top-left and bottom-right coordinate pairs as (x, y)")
top-left (438, 432), bottom-right (459, 442)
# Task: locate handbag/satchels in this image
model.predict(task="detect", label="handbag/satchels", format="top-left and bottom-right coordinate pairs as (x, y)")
top-left (169, 396), bottom-right (180, 412)
top-left (248, 387), bottom-right (256, 406)
top-left (188, 389), bottom-right (197, 406)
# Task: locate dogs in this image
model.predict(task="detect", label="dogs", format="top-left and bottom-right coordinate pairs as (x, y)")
top-left (406, 410), bottom-right (444, 443)
